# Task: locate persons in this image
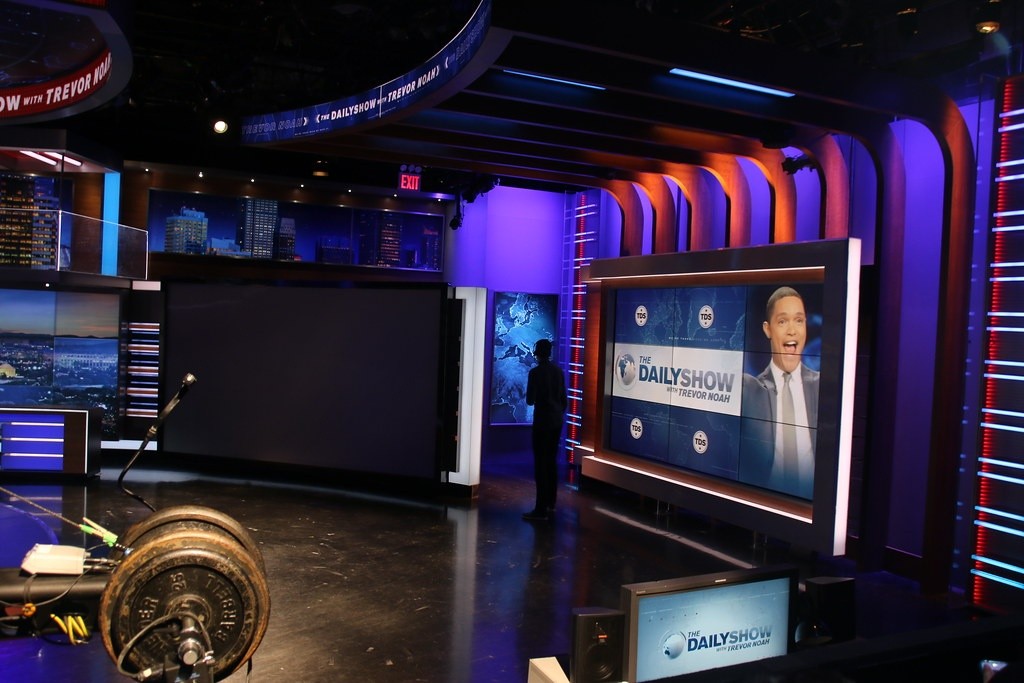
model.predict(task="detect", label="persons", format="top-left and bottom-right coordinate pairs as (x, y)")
top-left (520, 340), bottom-right (566, 523)
top-left (757, 286), bottom-right (821, 502)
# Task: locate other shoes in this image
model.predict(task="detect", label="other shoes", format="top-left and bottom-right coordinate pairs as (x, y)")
top-left (520, 504), bottom-right (556, 520)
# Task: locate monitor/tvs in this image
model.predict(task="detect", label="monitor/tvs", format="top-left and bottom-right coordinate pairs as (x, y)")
top-left (620, 566), bottom-right (800, 683)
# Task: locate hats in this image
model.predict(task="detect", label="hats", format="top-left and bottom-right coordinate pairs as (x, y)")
top-left (533, 340), bottom-right (552, 357)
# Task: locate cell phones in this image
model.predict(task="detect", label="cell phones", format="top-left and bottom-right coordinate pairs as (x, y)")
top-left (979, 659), bottom-right (1008, 674)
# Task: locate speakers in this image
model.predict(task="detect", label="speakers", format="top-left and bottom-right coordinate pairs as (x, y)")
top-left (570, 605), bottom-right (624, 683)
top-left (803, 576), bottom-right (858, 649)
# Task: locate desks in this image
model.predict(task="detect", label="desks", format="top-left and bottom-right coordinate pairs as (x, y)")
top-left (0, 405), bottom-right (103, 483)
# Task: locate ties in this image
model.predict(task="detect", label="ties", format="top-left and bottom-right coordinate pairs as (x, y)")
top-left (782, 373), bottom-right (799, 489)
